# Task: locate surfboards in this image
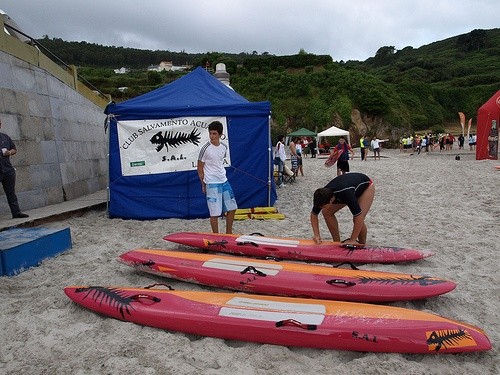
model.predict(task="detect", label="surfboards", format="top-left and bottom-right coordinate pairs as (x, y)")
top-left (64, 283), bottom-right (492, 353)
top-left (325, 150), bottom-right (344, 167)
top-left (164, 231), bottom-right (436, 265)
top-left (118, 247), bottom-right (456, 300)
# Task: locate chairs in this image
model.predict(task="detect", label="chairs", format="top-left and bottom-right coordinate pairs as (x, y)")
top-left (282, 167), bottom-right (295, 185)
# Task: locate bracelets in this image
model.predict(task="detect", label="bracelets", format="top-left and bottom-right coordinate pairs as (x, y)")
top-left (9, 151), bottom-right (11, 155)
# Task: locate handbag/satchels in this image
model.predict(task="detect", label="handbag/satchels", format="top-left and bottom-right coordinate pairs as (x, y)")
top-left (456, 155), bottom-right (460, 160)
top-left (274, 157), bottom-right (280, 165)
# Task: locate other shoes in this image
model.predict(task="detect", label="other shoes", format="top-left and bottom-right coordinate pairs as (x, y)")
top-left (279, 183), bottom-right (286, 188)
top-left (15, 213), bottom-right (29, 218)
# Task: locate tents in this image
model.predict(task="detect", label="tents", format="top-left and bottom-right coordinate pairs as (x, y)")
top-left (285, 126), bottom-right (351, 155)
top-left (476, 89), bottom-right (500, 160)
top-left (104, 66), bottom-right (276, 221)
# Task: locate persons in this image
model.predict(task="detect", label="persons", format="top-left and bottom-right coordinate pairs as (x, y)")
top-left (0, 120), bottom-right (29, 218)
top-left (198, 121), bottom-right (237, 234)
top-left (310, 173), bottom-right (375, 245)
top-left (273, 131), bottom-right (476, 188)
